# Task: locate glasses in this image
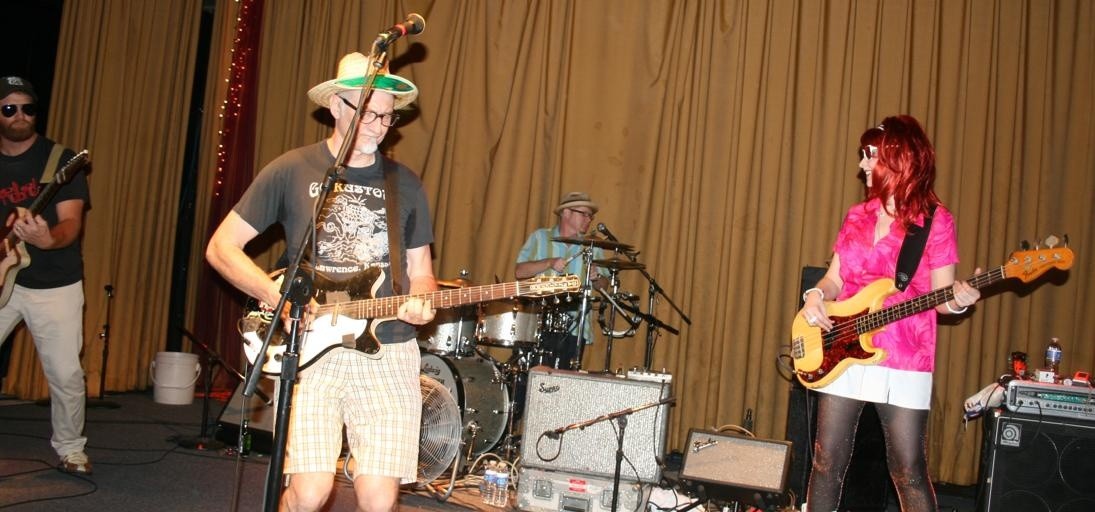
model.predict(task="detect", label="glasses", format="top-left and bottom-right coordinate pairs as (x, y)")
top-left (571, 209), bottom-right (589, 216)
top-left (863, 145), bottom-right (878, 159)
top-left (1, 104), bottom-right (37, 117)
top-left (338, 96), bottom-right (400, 127)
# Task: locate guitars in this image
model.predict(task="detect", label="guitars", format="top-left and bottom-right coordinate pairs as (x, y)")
top-left (791, 235), bottom-right (1074, 390)
top-left (239, 263), bottom-right (580, 376)
top-left (0, 150), bottom-right (88, 306)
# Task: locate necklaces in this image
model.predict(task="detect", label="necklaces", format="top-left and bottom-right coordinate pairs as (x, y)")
top-left (879, 207), bottom-right (895, 217)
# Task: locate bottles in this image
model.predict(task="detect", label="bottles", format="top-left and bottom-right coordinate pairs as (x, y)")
top-left (1043, 337), bottom-right (1063, 384)
top-left (480, 459), bottom-right (510, 508)
top-left (238, 418), bottom-right (252, 458)
top-left (1011, 351), bottom-right (1027, 377)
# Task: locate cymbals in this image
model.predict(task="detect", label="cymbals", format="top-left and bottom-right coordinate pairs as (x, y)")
top-left (582, 258), bottom-right (644, 270)
top-left (554, 235), bottom-right (638, 251)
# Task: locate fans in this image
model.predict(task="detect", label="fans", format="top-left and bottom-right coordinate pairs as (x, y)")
top-left (414, 375), bottom-right (463, 489)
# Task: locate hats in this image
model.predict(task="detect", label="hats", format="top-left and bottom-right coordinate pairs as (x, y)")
top-left (308, 52), bottom-right (418, 111)
top-left (0, 77), bottom-right (38, 101)
top-left (555, 192), bottom-right (598, 216)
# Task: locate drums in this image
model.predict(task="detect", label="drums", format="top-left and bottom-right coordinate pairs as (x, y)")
top-left (416, 276), bottom-right (478, 357)
top-left (420, 351), bottom-right (510, 459)
top-left (477, 291), bottom-right (540, 349)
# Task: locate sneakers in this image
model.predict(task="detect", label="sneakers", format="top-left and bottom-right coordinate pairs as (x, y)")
top-left (63, 452), bottom-right (91, 473)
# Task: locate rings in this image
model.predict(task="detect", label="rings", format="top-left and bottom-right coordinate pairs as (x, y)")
top-left (810, 317), bottom-right (816, 324)
top-left (16, 222), bottom-right (25, 231)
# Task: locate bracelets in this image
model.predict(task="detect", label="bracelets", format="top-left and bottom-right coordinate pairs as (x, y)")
top-left (945, 301), bottom-right (968, 315)
top-left (802, 287), bottom-right (824, 302)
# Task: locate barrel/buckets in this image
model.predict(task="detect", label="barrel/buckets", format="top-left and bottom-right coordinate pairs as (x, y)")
top-left (149, 351), bottom-right (202, 406)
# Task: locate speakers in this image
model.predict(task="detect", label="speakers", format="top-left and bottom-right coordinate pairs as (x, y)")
top-left (679, 428), bottom-right (794, 494)
top-left (520, 365), bottom-right (673, 483)
top-left (785, 271), bottom-right (903, 512)
top-left (973, 405), bottom-right (1095, 511)
top-left (217, 378), bottom-right (275, 456)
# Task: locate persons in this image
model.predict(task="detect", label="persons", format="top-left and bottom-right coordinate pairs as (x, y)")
top-left (204, 51), bottom-right (439, 512)
top-left (497, 191), bottom-right (612, 456)
top-left (800, 112), bottom-right (982, 512)
top-left (1, 76), bottom-right (95, 476)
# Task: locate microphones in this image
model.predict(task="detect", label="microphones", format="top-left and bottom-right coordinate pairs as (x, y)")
top-left (104, 285), bottom-right (114, 298)
top-left (597, 222), bottom-right (617, 242)
top-left (375, 12), bottom-right (425, 49)
